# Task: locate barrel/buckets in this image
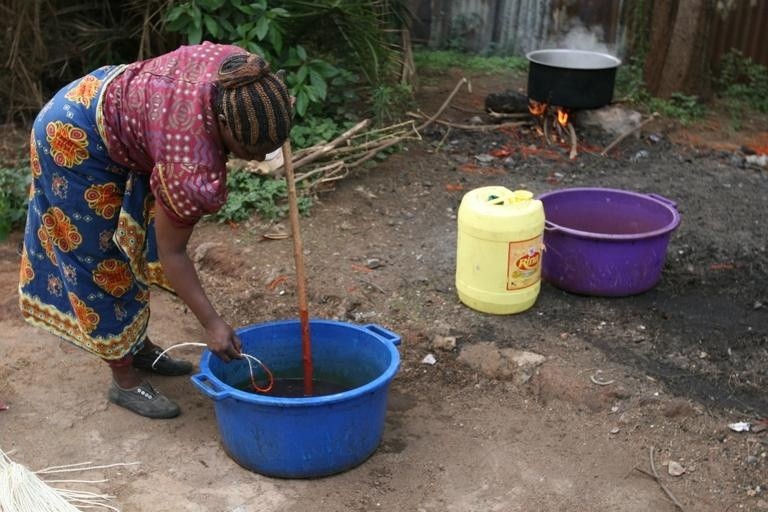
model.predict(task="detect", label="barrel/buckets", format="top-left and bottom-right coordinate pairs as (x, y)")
top-left (454, 185), bottom-right (545, 315)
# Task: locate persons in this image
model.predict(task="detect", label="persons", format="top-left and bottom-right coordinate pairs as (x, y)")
top-left (17, 41), bottom-right (296, 419)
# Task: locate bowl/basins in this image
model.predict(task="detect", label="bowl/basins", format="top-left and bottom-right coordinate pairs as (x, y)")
top-left (535, 186), bottom-right (682, 300)
top-left (189, 319), bottom-right (403, 481)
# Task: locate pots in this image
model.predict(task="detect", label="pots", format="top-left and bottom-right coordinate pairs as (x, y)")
top-left (523, 48), bottom-right (623, 111)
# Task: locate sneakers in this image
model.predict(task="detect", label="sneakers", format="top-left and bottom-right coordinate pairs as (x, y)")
top-left (135, 345), bottom-right (191, 375)
top-left (108, 378), bottom-right (180, 419)
